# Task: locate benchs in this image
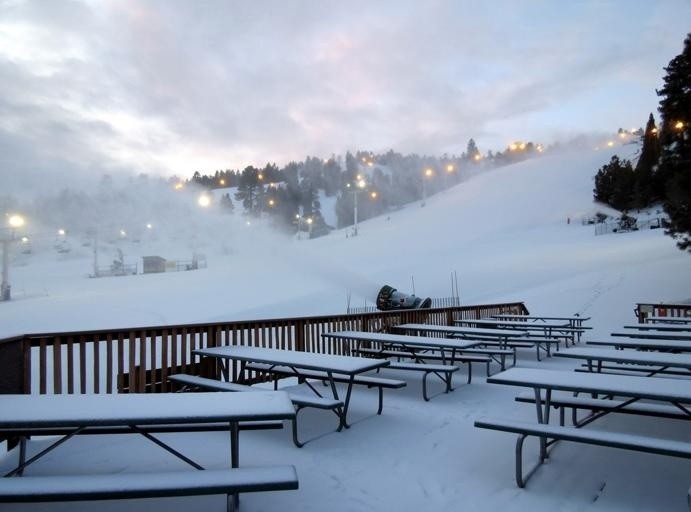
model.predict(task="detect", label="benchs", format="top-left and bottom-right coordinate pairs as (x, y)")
top-left (385, 342), bottom-right (515, 376)
top-left (166, 372), bottom-right (347, 449)
top-left (386, 362), bottom-right (460, 402)
top-left (244, 362), bottom-right (408, 417)
top-left (15, 418), bottom-right (283, 468)
top-left (351, 347), bottom-right (492, 384)
top-left (472, 315), bottom-right (691, 488)
top-left (0, 463), bottom-right (299, 511)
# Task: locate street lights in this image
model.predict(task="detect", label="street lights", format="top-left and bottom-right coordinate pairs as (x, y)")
top-left (443, 165), bottom-right (453, 191)
top-left (419, 168), bottom-right (433, 206)
top-left (345, 181), bottom-right (366, 236)
top-left (1, 215), bottom-right (24, 302)
top-left (296, 214), bottom-right (301, 240)
top-left (305, 217), bottom-right (312, 240)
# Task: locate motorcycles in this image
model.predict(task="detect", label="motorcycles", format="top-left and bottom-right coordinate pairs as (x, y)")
top-left (610, 208), bottom-right (639, 232)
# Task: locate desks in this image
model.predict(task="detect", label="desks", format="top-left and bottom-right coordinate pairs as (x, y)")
top-left (393, 323), bottom-right (529, 371)
top-left (190, 344), bottom-right (391, 432)
top-left (0, 389), bottom-right (296, 505)
top-left (320, 330), bottom-right (482, 393)
